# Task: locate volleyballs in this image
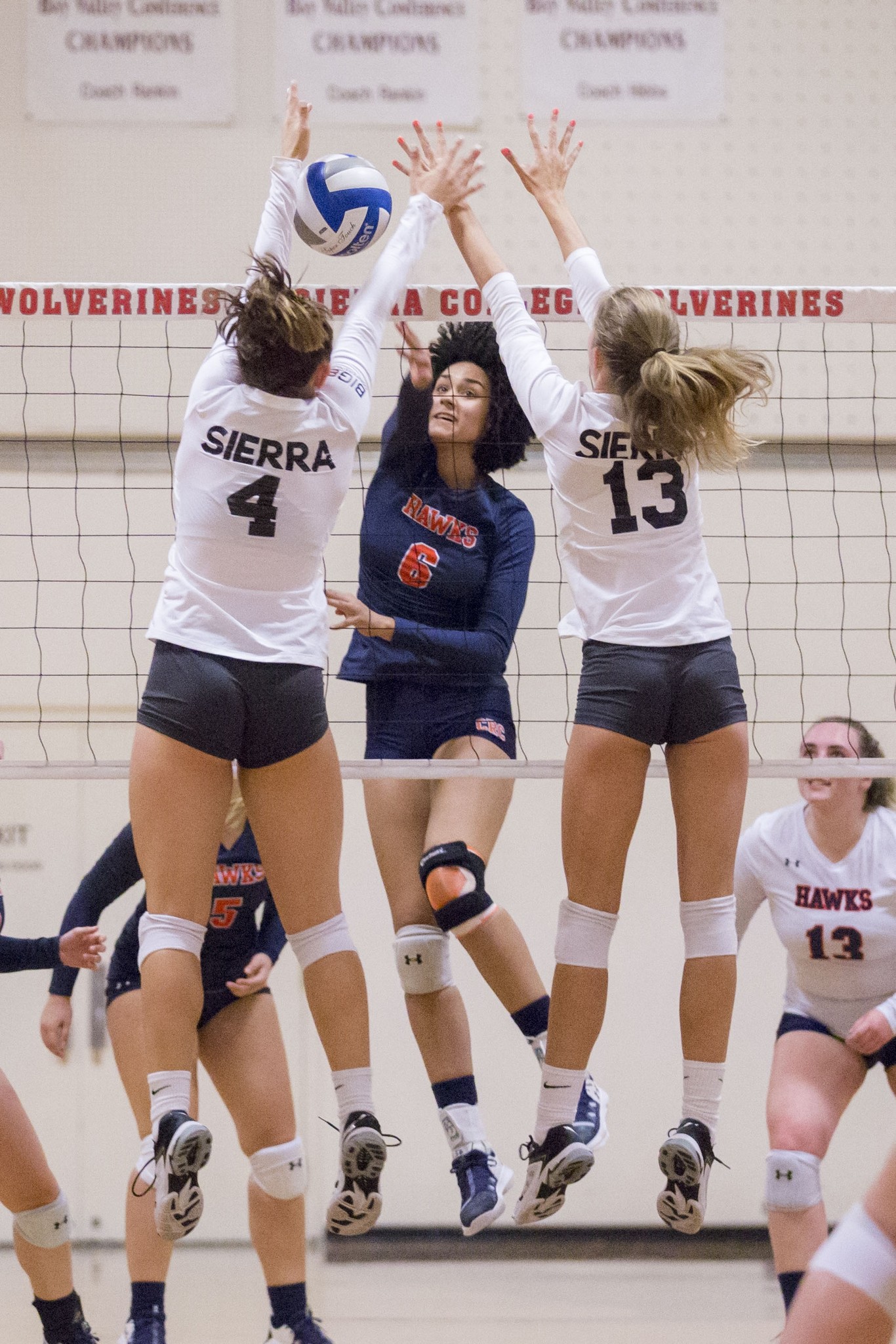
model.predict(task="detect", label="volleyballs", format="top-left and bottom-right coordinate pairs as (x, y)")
top-left (293, 153), bottom-right (393, 256)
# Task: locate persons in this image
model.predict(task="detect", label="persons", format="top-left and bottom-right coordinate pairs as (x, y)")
top-left (128, 85), bottom-right (484, 1242)
top-left (388, 109), bottom-right (770, 1240)
top-left (730, 718), bottom-right (896, 1344)
top-left (2, 759), bottom-right (336, 1344)
top-left (325, 316), bottom-right (606, 1236)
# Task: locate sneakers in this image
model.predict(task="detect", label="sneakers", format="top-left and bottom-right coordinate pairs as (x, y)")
top-left (510, 1121), bottom-right (594, 1226)
top-left (42, 1305), bottom-right (102, 1344)
top-left (317, 1110), bottom-right (401, 1237)
top-left (132, 1109), bottom-right (213, 1242)
top-left (656, 1116), bottom-right (731, 1237)
top-left (264, 1308), bottom-right (331, 1344)
top-left (572, 1072), bottom-right (612, 1149)
top-left (450, 1137), bottom-right (516, 1238)
top-left (124, 1302), bottom-right (166, 1344)
